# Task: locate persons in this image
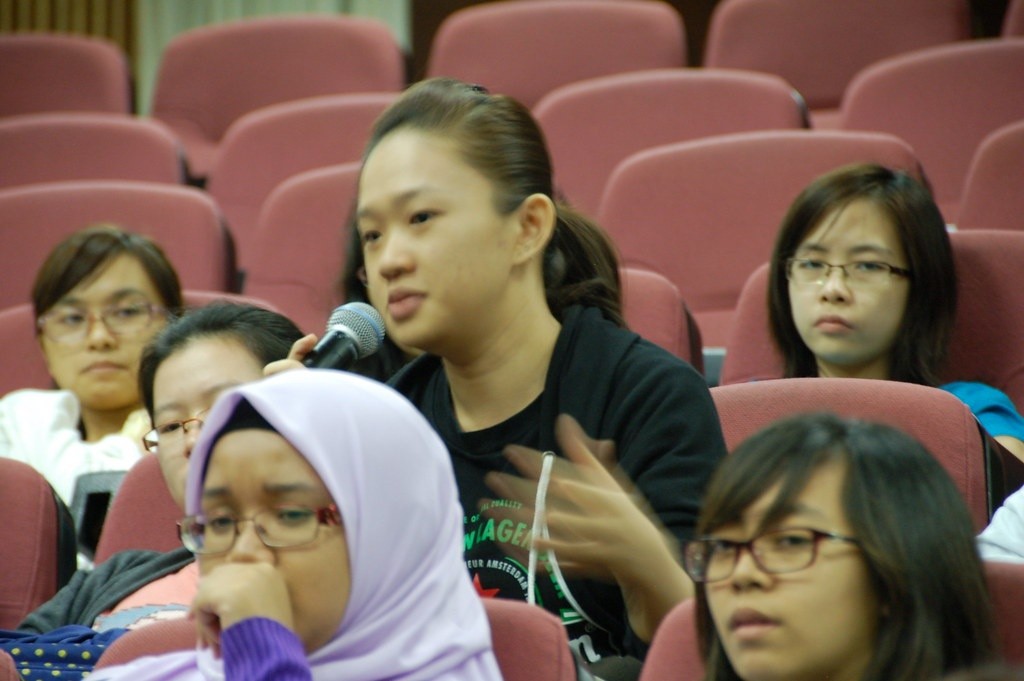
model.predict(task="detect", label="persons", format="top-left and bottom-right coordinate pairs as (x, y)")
top-left (80, 370), bottom-right (504, 681)
top-left (261, 79), bottom-right (728, 681)
top-left (767, 163), bottom-right (1024, 462)
top-left (0, 223), bottom-right (184, 499)
top-left (13, 297), bottom-right (305, 637)
top-left (343, 221), bottom-right (428, 381)
top-left (680, 411), bottom-right (1024, 681)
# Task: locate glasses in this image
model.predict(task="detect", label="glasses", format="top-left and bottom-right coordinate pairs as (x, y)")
top-left (177, 502), bottom-right (342, 556)
top-left (787, 256), bottom-right (913, 286)
top-left (142, 403), bottom-right (212, 453)
top-left (36, 301), bottom-right (169, 344)
top-left (682, 527), bottom-right (861, 582)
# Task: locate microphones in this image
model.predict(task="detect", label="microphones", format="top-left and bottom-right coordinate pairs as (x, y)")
top-left (303, 300), bottom-right (386, 371)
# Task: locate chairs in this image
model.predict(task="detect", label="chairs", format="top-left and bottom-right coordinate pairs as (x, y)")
top-left (0, 1), bottom-right (1023, 681)
